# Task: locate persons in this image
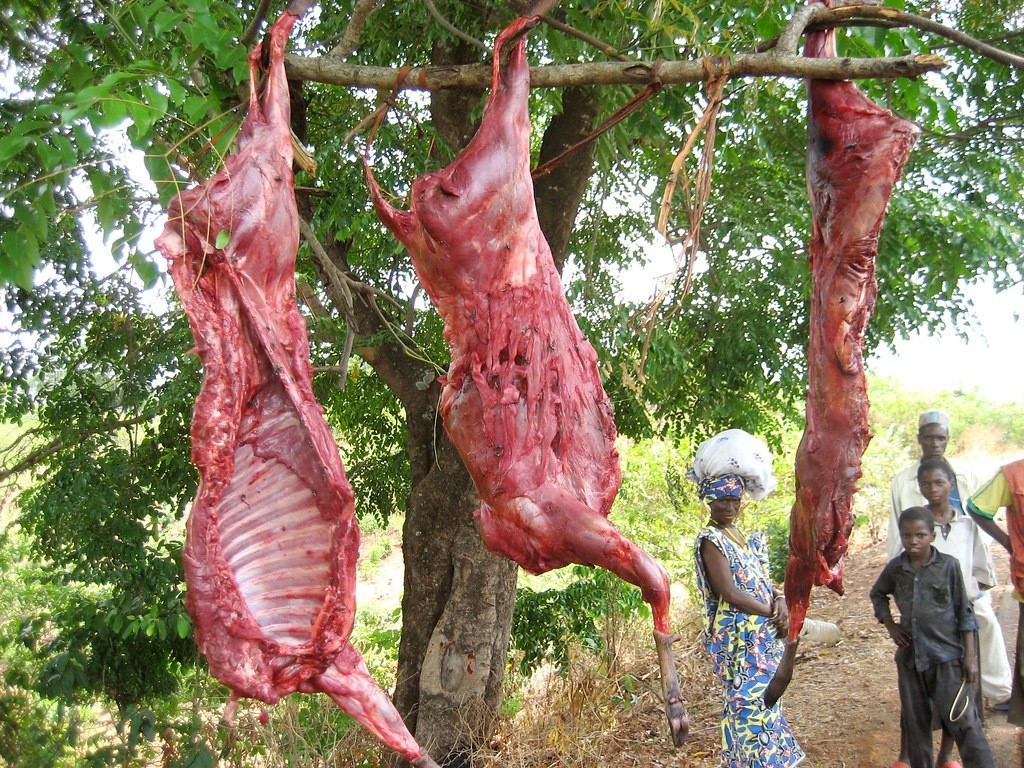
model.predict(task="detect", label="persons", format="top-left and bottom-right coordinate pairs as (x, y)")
top-left (967, 459), bottom-right (1024, 726)
top-left (888, 410), bottom-right (1013, 714)
top-left (890, 458), bottom-right (997, 768)
top-left (869, 506), bottom-right (995, 768)
top-left (686, 429), bottom-right (806, 768)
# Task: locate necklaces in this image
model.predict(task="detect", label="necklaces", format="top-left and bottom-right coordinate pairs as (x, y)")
top-left (708, 517), bottom-right (750, 553)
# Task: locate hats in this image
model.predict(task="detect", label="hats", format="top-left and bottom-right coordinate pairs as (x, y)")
top-left (690, 428), bottom-right (774, 506)
top-left (919, 412), bottom-right (950, 429)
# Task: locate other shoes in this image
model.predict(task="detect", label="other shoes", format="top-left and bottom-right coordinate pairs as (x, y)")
top-left (991, 697), bottom-right (1011, 711)
top-left (935, 754), bottom-right (964, 768)
top-left (891, 760), bottom-right (912, 768)
